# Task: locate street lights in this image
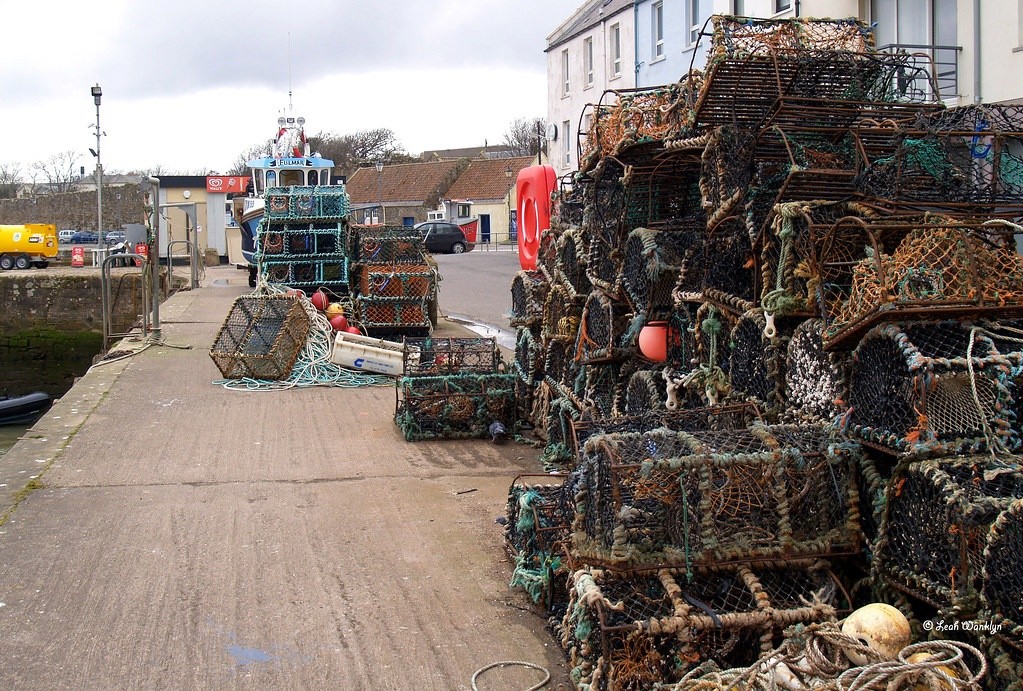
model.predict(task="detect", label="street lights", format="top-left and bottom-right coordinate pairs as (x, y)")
top-left (505, 163), bottom-right (514, 241)
top-left (89, 85), bottom-right (105, 248)
top-left (374, 161), bottom-right (384, 225)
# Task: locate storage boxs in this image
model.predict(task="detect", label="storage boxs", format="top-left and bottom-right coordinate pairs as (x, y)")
top-left (332, 330), bottom-right (421, 376)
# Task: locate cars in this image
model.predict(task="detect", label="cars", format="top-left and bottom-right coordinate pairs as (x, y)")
top-left (71, 230), bottom-right (93, 244)
top-left (58, 230), bottom-right (77, 245)
top-left (106, 230), bottom-right (127, 245)
top-left (90, 231), bottom-right (109, 243)
top-left (405, 221), bottom-right (468, 254)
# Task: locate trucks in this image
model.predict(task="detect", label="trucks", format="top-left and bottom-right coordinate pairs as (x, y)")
top-left (0, 222), bottom-right (58, 269)
